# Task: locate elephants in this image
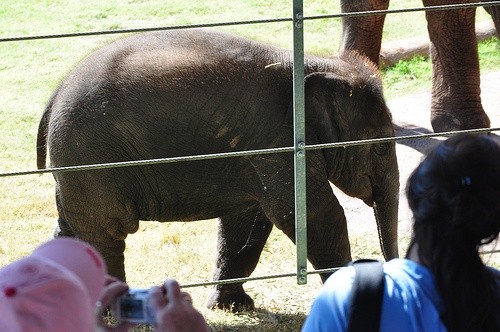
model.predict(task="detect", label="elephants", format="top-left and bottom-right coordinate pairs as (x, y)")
top-left (338, 1), bottom-right (500, 135)
top-left (32, 24), bottom-right (402, 313)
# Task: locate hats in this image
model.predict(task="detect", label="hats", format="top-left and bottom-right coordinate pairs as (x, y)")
top-left (0, 238), bottom-right (106, 332)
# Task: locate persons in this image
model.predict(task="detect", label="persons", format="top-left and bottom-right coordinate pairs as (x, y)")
top-left (0, 237), bottom-right (213, 332)
top-left (299, 133), bottom-right (500, 332)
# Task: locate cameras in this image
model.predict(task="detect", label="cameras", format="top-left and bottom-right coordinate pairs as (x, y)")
top-left (111, 288), bottom-right (157, 323)
